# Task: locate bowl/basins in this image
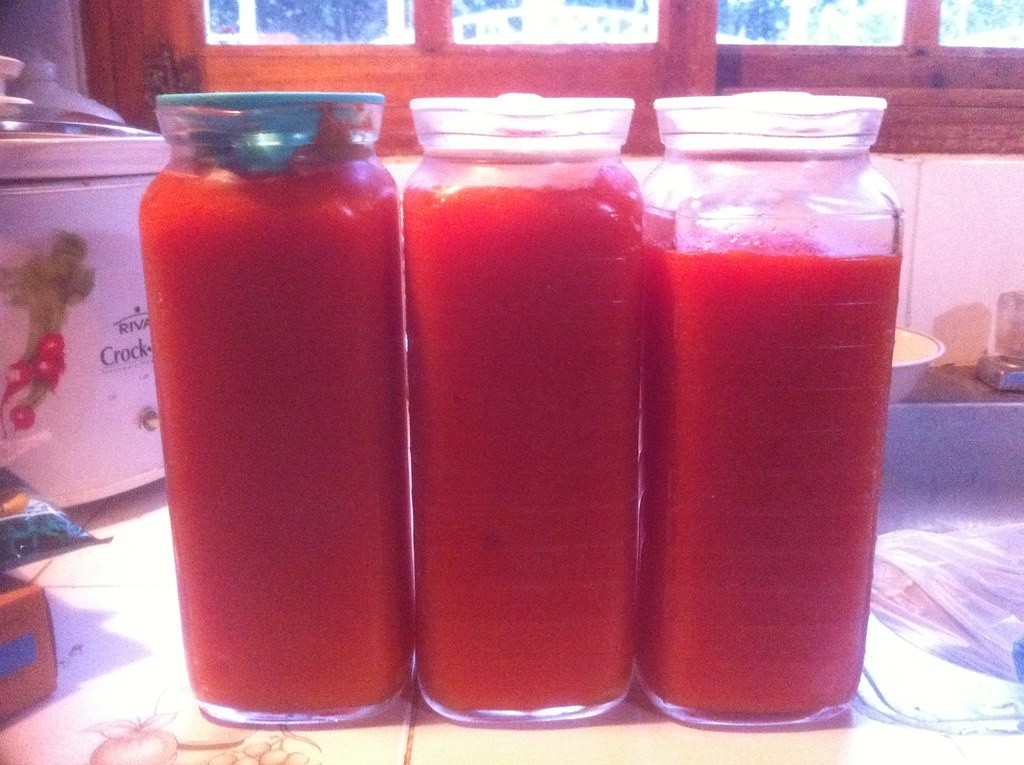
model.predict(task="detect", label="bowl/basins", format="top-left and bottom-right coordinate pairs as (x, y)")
top-left (887, 327), bottom-right (946, 404)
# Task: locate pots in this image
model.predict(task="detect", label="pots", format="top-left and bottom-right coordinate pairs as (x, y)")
top-left (2, 54), bottom-right (170, 511)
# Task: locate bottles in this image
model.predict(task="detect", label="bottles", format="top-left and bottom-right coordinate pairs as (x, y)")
top-left (133, 89), bottom-right (410, 724)
top-left (402, 94), bottom-right (640, 724)
top-left (639, 89), bottom-right (906, 725)
